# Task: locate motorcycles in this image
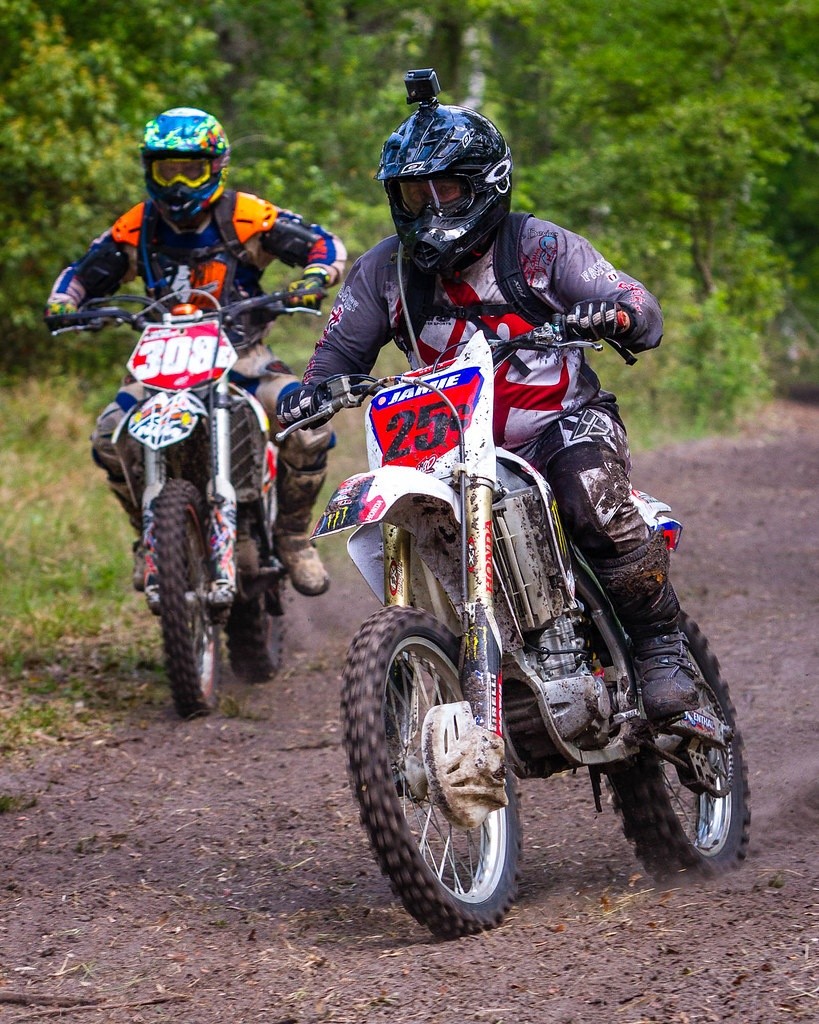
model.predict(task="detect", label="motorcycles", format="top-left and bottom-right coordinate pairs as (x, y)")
top-left (45, 289), bottom-right (327, 714)
top-left (272, 311), bottom-right (752, 940)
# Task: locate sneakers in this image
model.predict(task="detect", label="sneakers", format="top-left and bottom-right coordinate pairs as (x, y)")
top-left (105, 475), bottom-right (149, 593)
top-left (272, 429), bottom-right (336, 597)
top-left (597, 524), bottom-right (703, 722)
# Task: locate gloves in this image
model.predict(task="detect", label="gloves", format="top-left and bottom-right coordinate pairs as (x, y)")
top-left (275, 378), bottom-right (318, 430)
top-left (283, 267), bottom-right (330, 308)
top-left (566, 296), bottom-right (632, 344)
top-left (44, 301), bottom-right (78, 330)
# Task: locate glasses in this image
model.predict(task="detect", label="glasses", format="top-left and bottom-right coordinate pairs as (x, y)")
top-left (152, 160), bottom-right (212, 188)
top-left (391, 174), bottom-right (475, 217)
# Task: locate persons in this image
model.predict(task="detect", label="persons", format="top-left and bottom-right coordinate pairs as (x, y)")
top-left (278, 106), bottom-right (704, 717)
top-left (47, 107), bottom-right (348, 599)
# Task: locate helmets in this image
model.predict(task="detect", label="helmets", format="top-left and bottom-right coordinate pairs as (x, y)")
top-left (374, 67), bottom-right (514, 278)
top-left (137, 106), bottom-right (231, 222)
